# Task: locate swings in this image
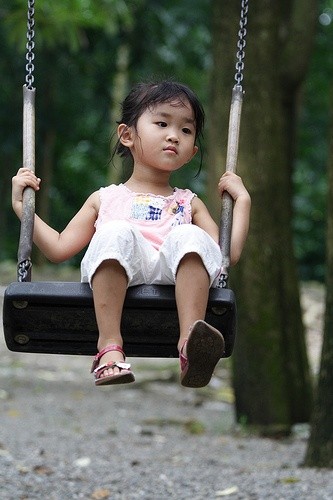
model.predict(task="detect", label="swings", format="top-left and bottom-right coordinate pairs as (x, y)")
top-left (3, 1), bottom-right (249, 358)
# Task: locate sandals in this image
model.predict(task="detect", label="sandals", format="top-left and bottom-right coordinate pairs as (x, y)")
top-left (91, 344), bottom-right (136, 386)
top-left (179, 319), bottom-right (225, 389)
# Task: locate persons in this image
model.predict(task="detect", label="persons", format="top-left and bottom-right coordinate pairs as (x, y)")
top-left (11, 80), bottom-right (252, 388)
